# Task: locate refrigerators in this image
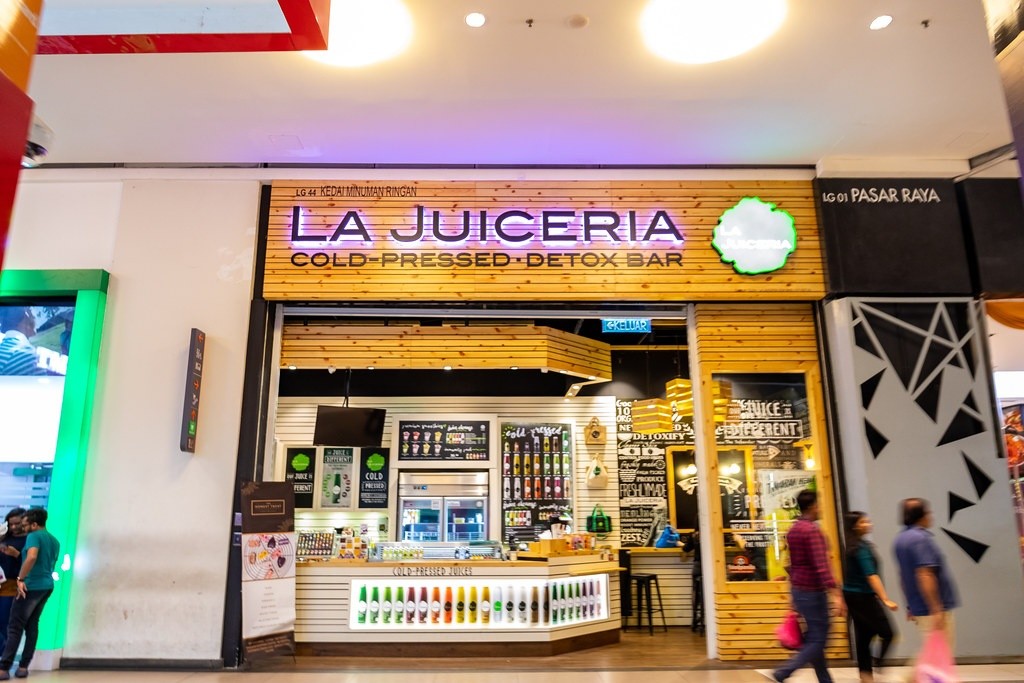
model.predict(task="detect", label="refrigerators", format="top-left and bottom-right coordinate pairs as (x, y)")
top-left (396, 472), bottom-right (489, 547)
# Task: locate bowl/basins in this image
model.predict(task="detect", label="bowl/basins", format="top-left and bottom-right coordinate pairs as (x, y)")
top-left (1005, 434), bottom-right (1024, 467)
top-left (456, 518), bottom-right (465, 523)
top-left (1004, 411), bottom-right (1023, 433)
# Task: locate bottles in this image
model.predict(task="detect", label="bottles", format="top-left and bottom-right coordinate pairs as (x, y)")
top-left (519, 587), bottom-right (527, 623)
top-left (469, 586), bottom-right (477, 623)
top-left (493, 586), bottom-right (502, 622)
top-left (418, 587), bottom-right (428, 623)
top-left (431, 586), bottom-right (440, 624)
top-left (504, 586), bottom-right (514, 623)
top-left (559, 584), bottom-right (566, 620)
top-left (395, 587), bottom-right (404, 623)
top-left (538, 507), bottom-right (557, 524)
top-left (405, 587), bottom-right (416, 623)
top-left (505, 426), bottom-right (569, 451)
top-left (443, 587), bottom-right (453, 624)
top-left (575, 580), bottom-right (600, 617)
top-left (505, 510), bottom-right (532, 526)
top-left (466, 453), bottom-right (486, 459)
top-left (567, 535), bottom-right (596, 549)
top-left (480, 586), bottom-right (490, 623)
top-left (446, 425), bottom-right (464, 445)
top-left (454, 546), bottom-right (495, 559)
top-left (383, 546), bottom-right (424, 560)
top-left (404, 509), bottom-right (421, 523)
top-left (456, 585), bottom-right (465, 623)
top-left (530, 586), bottom-right (539, 623)
top-left (567, 583), bottom-right (573, 619)
top-left (369, 586), bottom-right (379, 623)
top-left (358, 585), bottom-right (367, 623)
top-left (504, 477), bottom-right (570, 498)
top-left (551, 582), bottom-right (558, 622)
top-left (382, 587), bottom-right (392, 624)
top-left (503, 454), bottom-right (572, 475)
top-left (543, 583), bottom-right (550, 623)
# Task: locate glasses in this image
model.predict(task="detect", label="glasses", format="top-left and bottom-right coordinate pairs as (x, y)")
top-left (22, 523), bottom-right (30, 527)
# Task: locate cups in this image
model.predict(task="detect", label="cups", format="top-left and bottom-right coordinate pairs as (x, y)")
top-left (413, 432), bottom-right (420, 441)
top-left (404, 432), bottom-right (410, 441)
top-left (423, 445), bottom-right (430, 454)
top-left (435, 446), bottom-right (442, 454)
top-left (403, 445), bottom-right (409, 453)
top-left (435, 432), bottom-right (442, 441)
top-left (424, 432), bottom-right (431, 441)
top-left (413, 445), bottom-right (419, 453)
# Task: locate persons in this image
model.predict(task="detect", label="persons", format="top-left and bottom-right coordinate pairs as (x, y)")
top-left (0, 506), bottom-right (60, 680)
top-left (770, 488), bottom-right (848, 683)
top-left (681, 531), bottom-right (705, 638)
top-left (840, 510), bottom-right (899, 683)
top-left (893, 498), bottom-right (962, 683)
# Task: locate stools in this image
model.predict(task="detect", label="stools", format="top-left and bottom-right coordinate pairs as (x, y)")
top-left (624, 573), bottom-right (669, 633)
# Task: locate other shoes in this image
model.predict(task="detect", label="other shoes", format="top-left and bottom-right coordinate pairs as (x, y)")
top-left (0, 673), bottom-right (10, 680)
top-left (15, 668), bottom-right (28, 677)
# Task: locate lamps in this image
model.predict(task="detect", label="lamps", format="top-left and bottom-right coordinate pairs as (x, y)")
top-left (803, 444), bottom-right (816, 469)
top-left (729, 449), bottom-right (744, 475)
top-left (685, 450), bottom-right (698, 475)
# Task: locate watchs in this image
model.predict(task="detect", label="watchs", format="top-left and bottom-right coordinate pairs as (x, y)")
top-left (16, 577), bottom-right (24, 581)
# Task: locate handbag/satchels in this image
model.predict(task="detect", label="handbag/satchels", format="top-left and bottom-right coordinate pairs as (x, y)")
top-left (778, 614), bottom-right (805, 651)
top-left (584, 417), bottom-right (607, 445)
top-left (586, 453), bottom-right (608, 488)
top-left (587, 504), bottom-right (612, 532)
top-left (655, 525), bottom-right (680, 548)
